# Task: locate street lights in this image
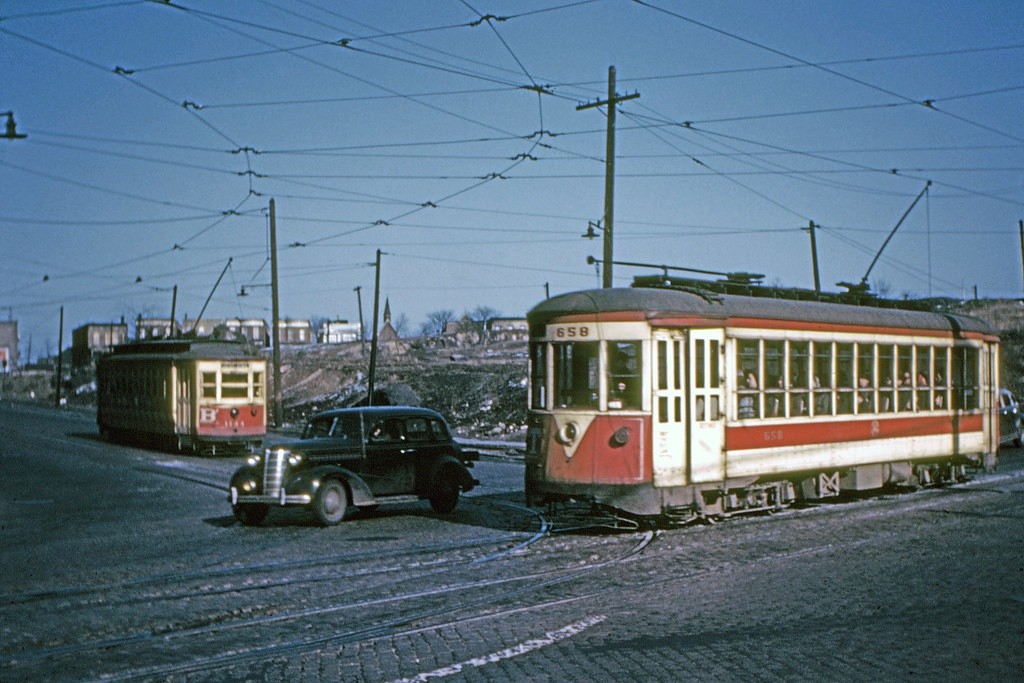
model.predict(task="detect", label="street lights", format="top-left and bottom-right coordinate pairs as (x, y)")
top-left (575, 65), bottom-right (641, 289)
top-left (580, 221), bottom-right (612, 290)
top-left (236, 200), bottom-right (283, 429)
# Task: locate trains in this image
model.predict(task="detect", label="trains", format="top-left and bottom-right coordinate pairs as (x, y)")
top-left (522, 178), bottom-right (1002, 528)
top-left (96, 257), bottom-right (269, 457)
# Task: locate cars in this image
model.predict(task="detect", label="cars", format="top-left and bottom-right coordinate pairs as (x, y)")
top-left (227, 406), bottom-right (480, 527)
top-left (973, 387), bottom-right (1024, 449)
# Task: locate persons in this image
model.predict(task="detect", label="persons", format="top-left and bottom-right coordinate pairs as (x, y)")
top-left (612, 351), bottom-right (636, 389)
top-left (736, 368), bottom-right (957, 419)
